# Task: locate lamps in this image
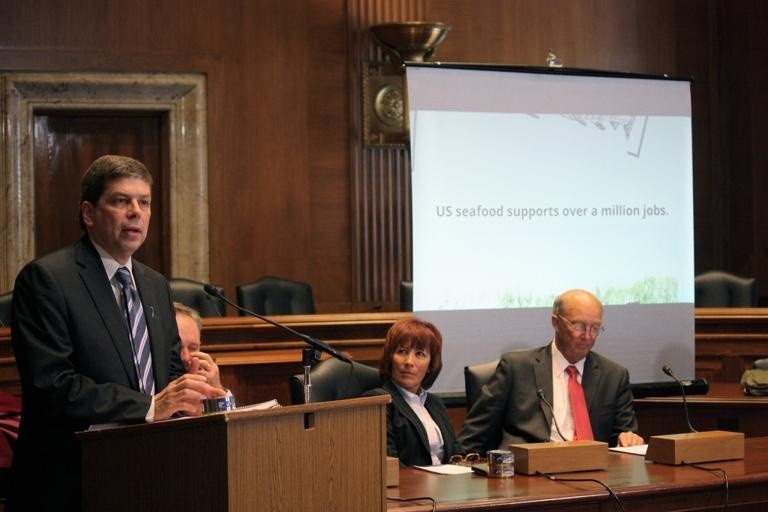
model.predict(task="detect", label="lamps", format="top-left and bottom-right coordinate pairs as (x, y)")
top-left (363, 22), bottom-right (452, 148)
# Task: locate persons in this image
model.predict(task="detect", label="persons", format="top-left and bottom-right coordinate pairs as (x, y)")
top-left (355, 318), bottom-right (469, 470)
top-left (170, 300), bottom-right (232, 397)
top-left (456, 287), bottom-right (645, 462)
top-left (3, 154), bottom-right (229, 511)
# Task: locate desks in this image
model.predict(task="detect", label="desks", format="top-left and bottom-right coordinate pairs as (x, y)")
top-left (386, 436), bottom-right (768, 511)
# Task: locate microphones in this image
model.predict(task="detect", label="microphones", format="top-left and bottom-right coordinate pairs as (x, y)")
top-left (205, 285), bottom-right (355, 373)
top-left (662, 365), bottom-right (698, 433)
top-left (535, 388), bottom-right (567, 442)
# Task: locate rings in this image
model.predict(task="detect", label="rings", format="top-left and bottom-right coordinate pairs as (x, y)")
top-left (206, 363), bottom-right (209, 370)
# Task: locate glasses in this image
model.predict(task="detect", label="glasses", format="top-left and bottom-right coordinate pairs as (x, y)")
top-left (558, 314), bottom-right (604, 334)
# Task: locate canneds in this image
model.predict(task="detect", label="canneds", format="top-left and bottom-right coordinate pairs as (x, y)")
top-left (486, 449), bottom-right (515, 477)
top-left (200, 396), bottom-right (231, 414)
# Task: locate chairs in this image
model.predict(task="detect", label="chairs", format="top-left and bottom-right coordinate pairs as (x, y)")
top-left (235, 275), bottom-right (315, 315)
top-left (168, 278), bottom-right (226, 317)
top-left (695, 270), bottom-right (758, 308)
top-left (464, 358), bottom-right (500, 412)
top-left (400, 280), bottom-right (414, 312)
top-left (289, 356), bottom-right (385, 403)
top-left (753, 359), bottom-right (768, 369)
top-left (1, 291), bottom-right (14, 325)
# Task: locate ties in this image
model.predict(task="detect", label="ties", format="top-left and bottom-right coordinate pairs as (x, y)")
top-left (115, 267), bottom-right (155, 396)
top-left (566, 366), bottom-right (594, 440)
